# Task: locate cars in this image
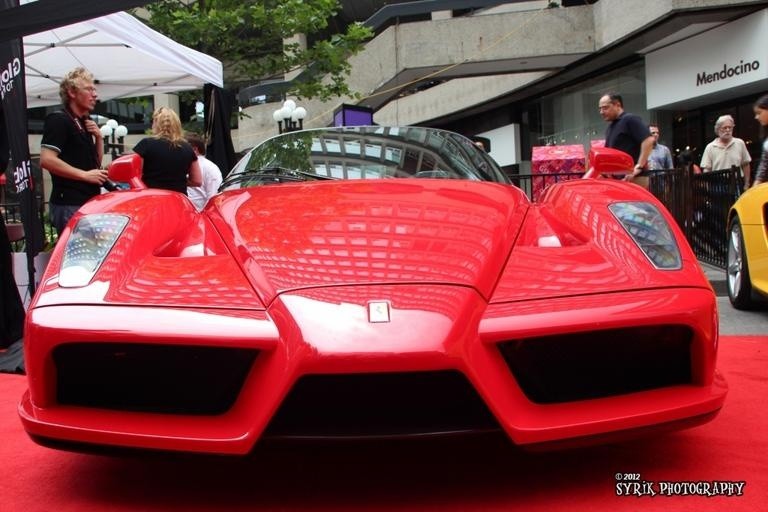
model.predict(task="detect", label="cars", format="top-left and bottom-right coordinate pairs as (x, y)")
top-left (725, 181), bottom-right (768, 312)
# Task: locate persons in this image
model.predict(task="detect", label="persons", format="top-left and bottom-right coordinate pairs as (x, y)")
top-left (132, 105), bottom-right (223, 213)
top-left (752, 95), bottom-right (768, 187)
top-left (40, 66), bottom-right (108, 239)
top-left (677, 150), bottom-right (701, 174)
top-left (700, 115), bottom-right (752, 209)
top-left (599, 91), bottom-right (674, 207)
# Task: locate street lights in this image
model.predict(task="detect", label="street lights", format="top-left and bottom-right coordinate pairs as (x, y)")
top-left (273, 99), bottom-right (307, 134)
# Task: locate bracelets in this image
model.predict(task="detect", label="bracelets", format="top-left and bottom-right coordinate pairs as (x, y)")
top-left (635, 164), bottom-right (644, 169)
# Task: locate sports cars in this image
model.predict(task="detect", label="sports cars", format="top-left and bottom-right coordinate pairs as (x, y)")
top-left (17, 125), bottom-right (730, 462)
